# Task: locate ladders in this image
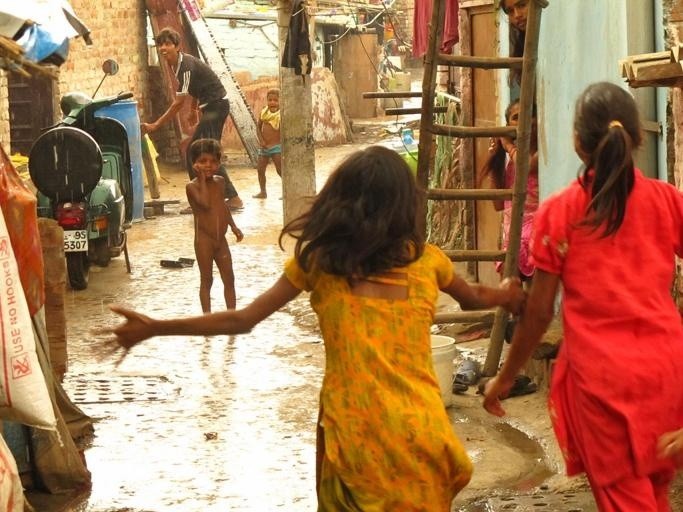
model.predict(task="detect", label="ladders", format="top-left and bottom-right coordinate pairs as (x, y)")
top-left (415, 0), bottom-right (545, 377)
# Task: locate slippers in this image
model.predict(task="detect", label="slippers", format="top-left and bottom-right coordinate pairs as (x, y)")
top-left (453, 360), bottom-right (537, 400)
top-left (180, 207), bottom-right (193, 214)
top-left (226, 203), bottom-right (245, 210)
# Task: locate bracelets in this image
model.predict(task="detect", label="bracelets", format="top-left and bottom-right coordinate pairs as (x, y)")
top-left (508, 146), bottom-right (517, 159)
top-left (486, 176), bottom-right (498, 184)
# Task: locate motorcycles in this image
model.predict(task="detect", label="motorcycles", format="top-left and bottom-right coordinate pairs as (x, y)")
top-left (27, 59), bottom-right (133, 291)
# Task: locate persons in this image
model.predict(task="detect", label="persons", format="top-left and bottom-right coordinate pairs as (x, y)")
top-left (89, 145), bottom-right (531, 511)
top-left (249, 87), bottom-right (282, 202)
top-left (137, 25), bottom-right (244, 214)
top-left (498, 1), bottom-right (535, 92)
top-left (184, 136), bottom-right (244, 313)
top-left (480, 93), bottom-right (537, 289)
top-left (480, 81), bottom-right (682, 512)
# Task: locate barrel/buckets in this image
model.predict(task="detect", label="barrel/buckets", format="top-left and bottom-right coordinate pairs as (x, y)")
top-left (392, 138), bottom-right (419, 151)
top-left (398, 152), bottom-right (419, 176)
top-left (429, 334), bottom-right (458, 409)
